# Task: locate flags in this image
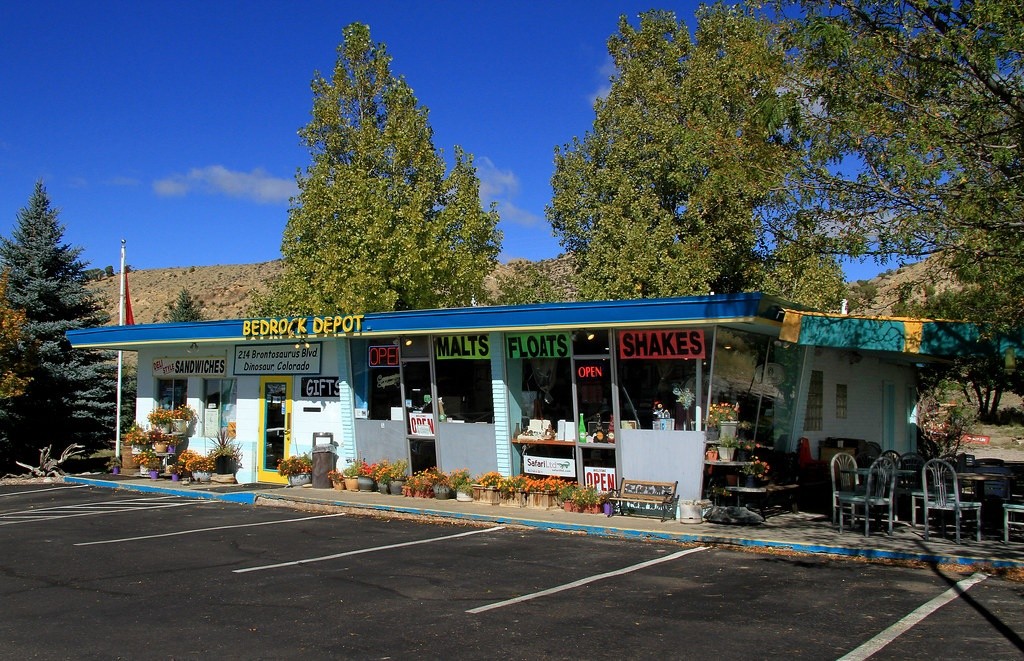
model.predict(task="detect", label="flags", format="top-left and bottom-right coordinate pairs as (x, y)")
top-left (126, 268), bottom-right (135, 325)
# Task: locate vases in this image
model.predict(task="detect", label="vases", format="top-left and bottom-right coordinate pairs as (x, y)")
top-left (343, 476), bottom-right (359, 492)
top-left (357, 476), bottom-right (374, 492)
top-left (122, 445), bottom-right (146, 469)
top-left (433, 484), bottom-right (451, 500)
top-left (140, 464), bottom-right (152, 474)
top-left (149, 470), bottom-right (158, 479)
top-left (332, 479), bottom-right (344, 490)
top-left (471, 484), bottom-right (559, 511)
top-left (215, 455), bottom-right (233, 474)
top-left (686, 409), bottom-right (690, 431)
top-left (192, 471), bottom-right (212, 482)
top-left (183, 469), bottom-right (192, 478)
top-left (737, 449), bottom-right (752, 462)
top-left (172, 473), bottom-right (179, 481)
top-left (131, 444), bottom-right (144, 454)
top-left (286, 474), bottom-right (311, 486)
top-left (402, 485), bottom-right (434, 498)
top-left (157, 423), bottom-right (170, 434)
top-left (172, 419), bottom-right (186, 433)
top-left (603, 503), bottom-right (615, 514)
top-left (745, 474), bottom-right (759, 488)
top-left (389, 478), bottom-right (406, 494)
top-left (378, 481), bottom-right (389, 494)
top-left (168, 445), bottom-right (176, 453)
top-left (720, 421), bottom-right (740, 438)
top-left (154, 441), bottom-right (166, 453)
top-left (564, 502), bottom-right (602, 514)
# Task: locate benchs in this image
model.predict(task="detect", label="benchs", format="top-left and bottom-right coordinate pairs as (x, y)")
top-left (607, 478), bottom-right (679, 522)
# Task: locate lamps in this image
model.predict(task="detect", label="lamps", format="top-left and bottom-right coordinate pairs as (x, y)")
top-left (585, 330), bottom-right (594, 340)
top-left (403, 337), bottom-right (412, 345)
top-left (393, 337), bottom-right (401, 344)
top-left (303, 340), bottom-right (309, 348)
top-left (294, 340), bottom-right (301, 348)
top-left (185, 344), bottom-right (194, 353)
top-left (569, 330), bottom-right (579, 341)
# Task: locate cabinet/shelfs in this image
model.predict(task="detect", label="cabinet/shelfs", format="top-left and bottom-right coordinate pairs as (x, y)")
top-left (703, 441), bottom-right (799, 522)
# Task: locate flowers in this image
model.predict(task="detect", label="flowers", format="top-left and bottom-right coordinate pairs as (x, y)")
top-left (121, 403), bottom-right (243, 476)
top-left (672, 380), bottom-right (696, 410)
top-left (739, 455), bottom-right (770, 478)
top-left (277, 452), bottom-right (312, 476)
top-left (327, 458), bottom-right (613, 510)
top-left (702, 402), bottom-right (740, 433)
top-left (732, 431), bottom-right (763, 452)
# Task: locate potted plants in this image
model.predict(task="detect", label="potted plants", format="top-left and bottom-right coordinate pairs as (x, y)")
top-left (104, 455), bottom-right (121, 474)
top-left (706, 445), bottom-right (718, 462)
top-left (717, 436), bottom-right (740, 462)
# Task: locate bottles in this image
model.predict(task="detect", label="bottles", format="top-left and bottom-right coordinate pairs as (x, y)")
top-left (593, 413), bottom-right (608, 443)
top-left (664, 410), bottom-right (670, 418)
top-left (514, 423), bottom-right (521, 439)
top-left (579, 413), bottom-right (586, 442)
top-left (607, 415), bottom-right (615, 443)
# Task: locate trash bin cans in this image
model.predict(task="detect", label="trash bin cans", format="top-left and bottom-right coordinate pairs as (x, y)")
top-left (975, 459), bottom-right (1006, 501)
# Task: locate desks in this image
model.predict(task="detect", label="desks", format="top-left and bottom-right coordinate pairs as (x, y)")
top-left (152, 452), bottom-right (175, 476)
top-left (840, 468), bottom-right (917, 532)
top-left (945, 471), bottom-right (1012, 540)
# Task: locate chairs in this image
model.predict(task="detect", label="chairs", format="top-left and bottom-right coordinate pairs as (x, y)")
top-left (830, 450), bottom-right (1024, 545)
top-left (799, 437), bottom-right (828, 469)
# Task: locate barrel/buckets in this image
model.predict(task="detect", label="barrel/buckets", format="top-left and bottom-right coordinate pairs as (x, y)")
top-left (680, 500), bottom-right (702, 524)
top-left (311, 452), bottom-right (333, 489)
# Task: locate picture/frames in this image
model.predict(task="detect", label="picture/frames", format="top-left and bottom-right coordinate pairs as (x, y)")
top-left (457, 491), bottom-right (473, 502)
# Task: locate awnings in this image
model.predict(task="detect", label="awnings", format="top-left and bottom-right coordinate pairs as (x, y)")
top-left (778, 307), bottom-right (1024, 374)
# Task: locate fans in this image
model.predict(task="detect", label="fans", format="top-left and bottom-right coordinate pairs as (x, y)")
top-left (755, 362), bottom-right (786, 385)
